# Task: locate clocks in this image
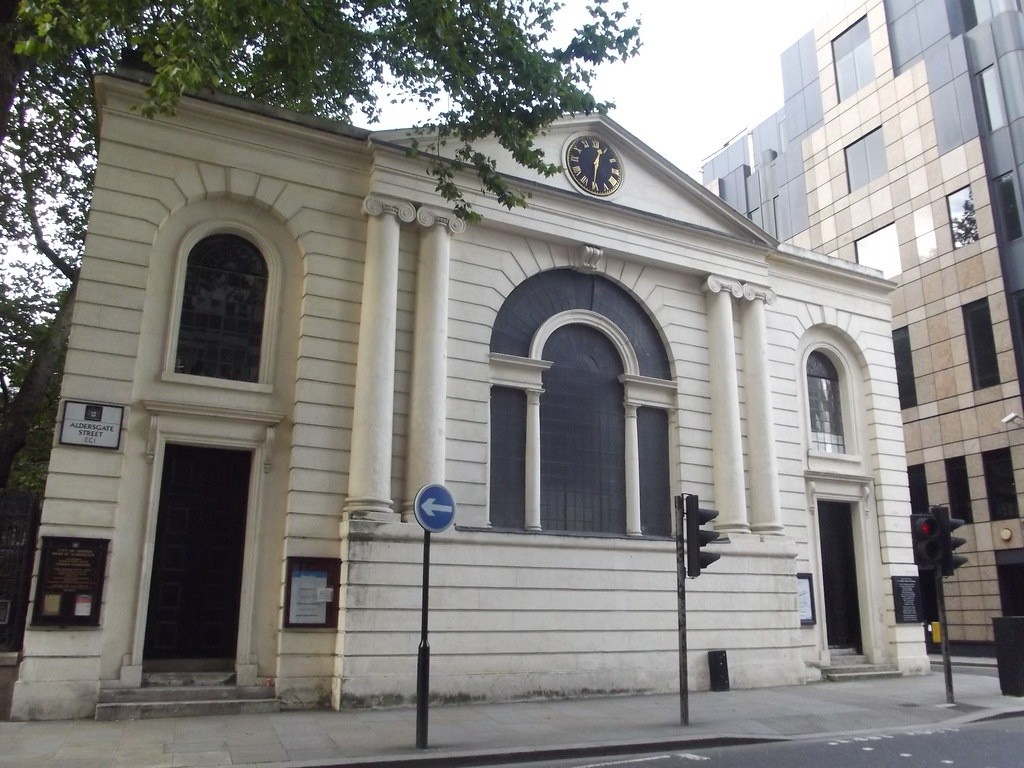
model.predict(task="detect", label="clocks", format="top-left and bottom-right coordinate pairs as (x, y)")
top-left (562, 131), bottom-right (627, 201)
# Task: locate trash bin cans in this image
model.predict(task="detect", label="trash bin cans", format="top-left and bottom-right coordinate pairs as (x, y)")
top-left (707, 650), bottom-right (730, 691)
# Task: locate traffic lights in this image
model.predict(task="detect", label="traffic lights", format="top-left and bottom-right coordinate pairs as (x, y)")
top-left (931, 506), bottom-right (969, 576)
top-left (686, 495), bottom-right (720, 578)
top-left (910, 514), bottom-right (942, 570)
